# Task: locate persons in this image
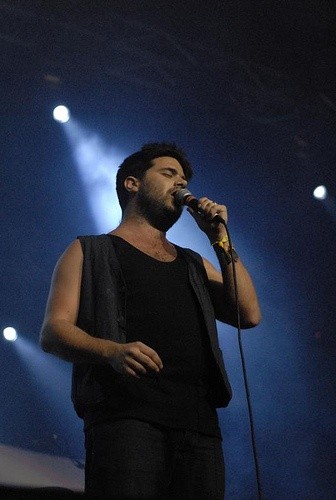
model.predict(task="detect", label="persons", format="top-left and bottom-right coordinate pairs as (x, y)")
top-left (40, 143), bottom-right (261, 500)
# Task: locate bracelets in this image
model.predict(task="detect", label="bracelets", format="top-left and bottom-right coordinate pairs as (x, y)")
top-left (211, 236), bottom-right (228, 247)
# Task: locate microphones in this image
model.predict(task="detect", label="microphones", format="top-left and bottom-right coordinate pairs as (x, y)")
top-left (175, 188), bottom-right (225, 224)
